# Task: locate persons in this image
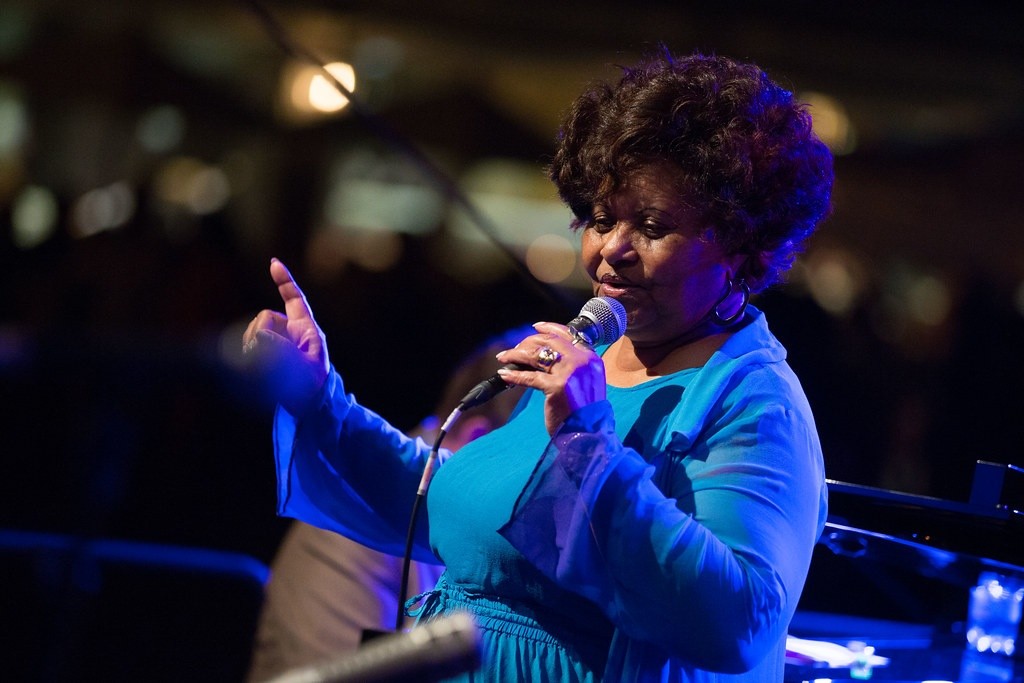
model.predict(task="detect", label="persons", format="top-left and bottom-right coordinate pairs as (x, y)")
top-left (240, 56), bottom-right (834, 683)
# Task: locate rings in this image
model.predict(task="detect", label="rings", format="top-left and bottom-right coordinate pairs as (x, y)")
top-left (567, 328), bottom-right (593, 351)
top-left (537, 347), bottom-right (563, 374)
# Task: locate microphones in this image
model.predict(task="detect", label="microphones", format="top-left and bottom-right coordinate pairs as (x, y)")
top-left (270, 613), bottom-right (483, 683)
top-left (460, 296), bottom-right (627, 411)
